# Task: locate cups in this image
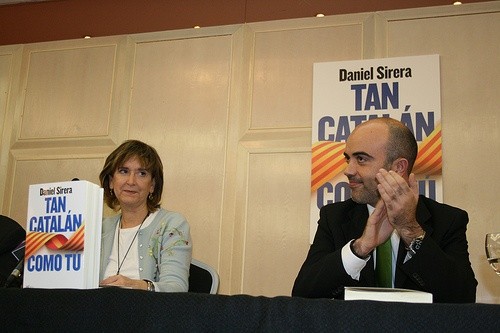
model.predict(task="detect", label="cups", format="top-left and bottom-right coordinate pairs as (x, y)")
top-left (485, 233), bottom-right (500, 275)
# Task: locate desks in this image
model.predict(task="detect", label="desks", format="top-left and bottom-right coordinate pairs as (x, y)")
top-left (0, 286), bottom-right (500, 333)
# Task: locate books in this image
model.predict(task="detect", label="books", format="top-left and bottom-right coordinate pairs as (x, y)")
top-left (344, 286), bottom-right (434, 303)
top-left (23, 180), bottom-right (105, 289)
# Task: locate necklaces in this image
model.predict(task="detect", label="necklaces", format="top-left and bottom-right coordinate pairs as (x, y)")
top-left (115, 211), bottom-right (150, 274)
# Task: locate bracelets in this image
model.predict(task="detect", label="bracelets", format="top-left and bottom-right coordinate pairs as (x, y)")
top-left (146, 280), bottom-right (155, 291)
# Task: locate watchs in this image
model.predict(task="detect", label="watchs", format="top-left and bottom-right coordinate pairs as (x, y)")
top-left (406, 232), bottom-right (425, 255)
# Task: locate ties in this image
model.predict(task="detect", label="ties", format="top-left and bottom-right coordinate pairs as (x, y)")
top-left (375, 238), bottom-right (392, 288)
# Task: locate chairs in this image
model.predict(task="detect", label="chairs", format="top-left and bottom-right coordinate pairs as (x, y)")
top-left (185, 260), bottom-right (220, 296)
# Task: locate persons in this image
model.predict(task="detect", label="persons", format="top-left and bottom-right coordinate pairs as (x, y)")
top-left (0, 215), bottom-right (26, 288)
top-left (99, 141), bottom-right (192, 293)
top-left (291, 118), bottom-right (478, 303)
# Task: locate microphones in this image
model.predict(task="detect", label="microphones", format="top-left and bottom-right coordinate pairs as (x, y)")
top-left (5, 258), bottom-right (24, 287)
